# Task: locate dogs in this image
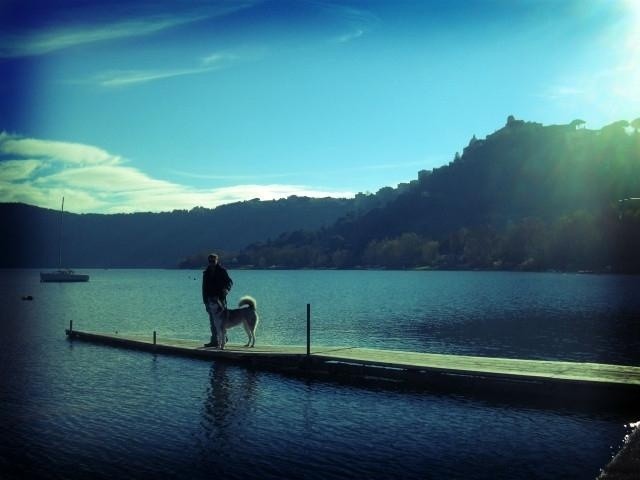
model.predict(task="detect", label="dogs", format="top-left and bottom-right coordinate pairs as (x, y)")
top-left (206, 295), bottom-right (258, 350)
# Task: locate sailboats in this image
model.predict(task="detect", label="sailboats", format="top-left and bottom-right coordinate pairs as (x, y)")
top-left (39, 196), bottom-right (91, 284)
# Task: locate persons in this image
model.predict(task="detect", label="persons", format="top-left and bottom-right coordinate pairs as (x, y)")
top-left (202, 254), bottom-right (233, 348)
